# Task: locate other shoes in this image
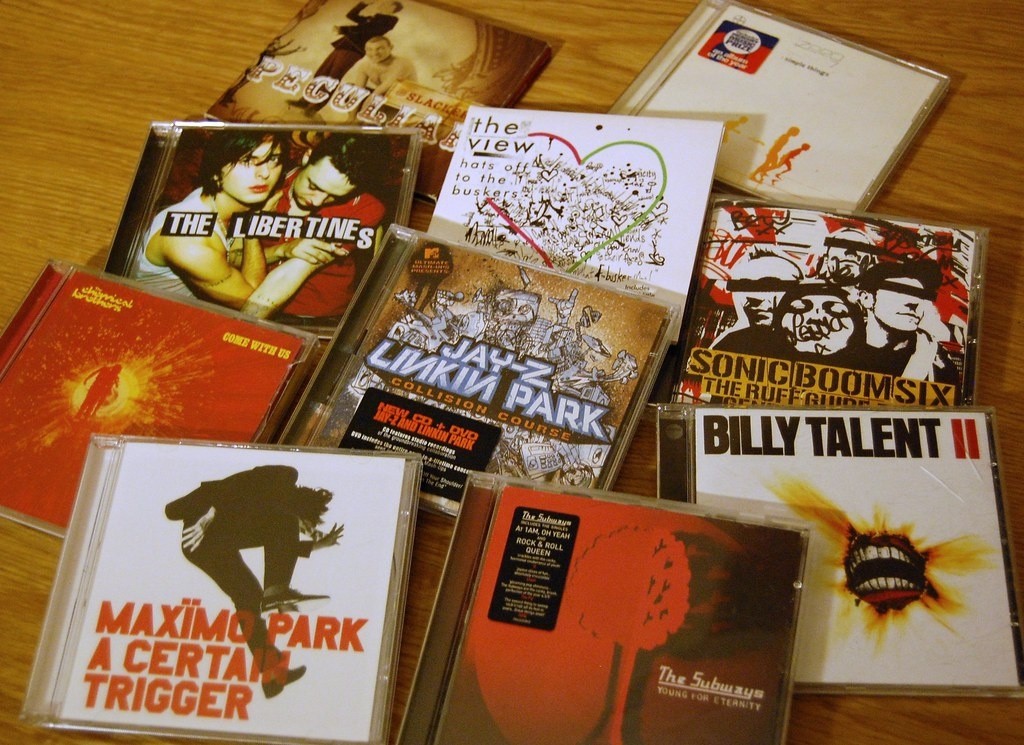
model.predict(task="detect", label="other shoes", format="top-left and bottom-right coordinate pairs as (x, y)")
top-left (260, 591), bottom-right (330, 616)
top-left (302, 108), bottom-right (314, 119)
top-left (261, 665), bottom-right (308, 697)
top-left (285, 98), bottom-right (306, 109)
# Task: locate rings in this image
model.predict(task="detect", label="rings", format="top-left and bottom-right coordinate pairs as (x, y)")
top-left (331, 243), bottom-right (336, 254)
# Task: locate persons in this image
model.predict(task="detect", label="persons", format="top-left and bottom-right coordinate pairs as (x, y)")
top-left (285, 0), bottom-right (421, 128)
top-left (165, 464), bottom-right (345, 700)
top-left (129, 129), bottom-right (386, 320)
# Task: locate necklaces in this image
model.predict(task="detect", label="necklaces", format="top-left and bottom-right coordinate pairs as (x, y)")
top-left (210, 197), bottom-right (228, 235)
